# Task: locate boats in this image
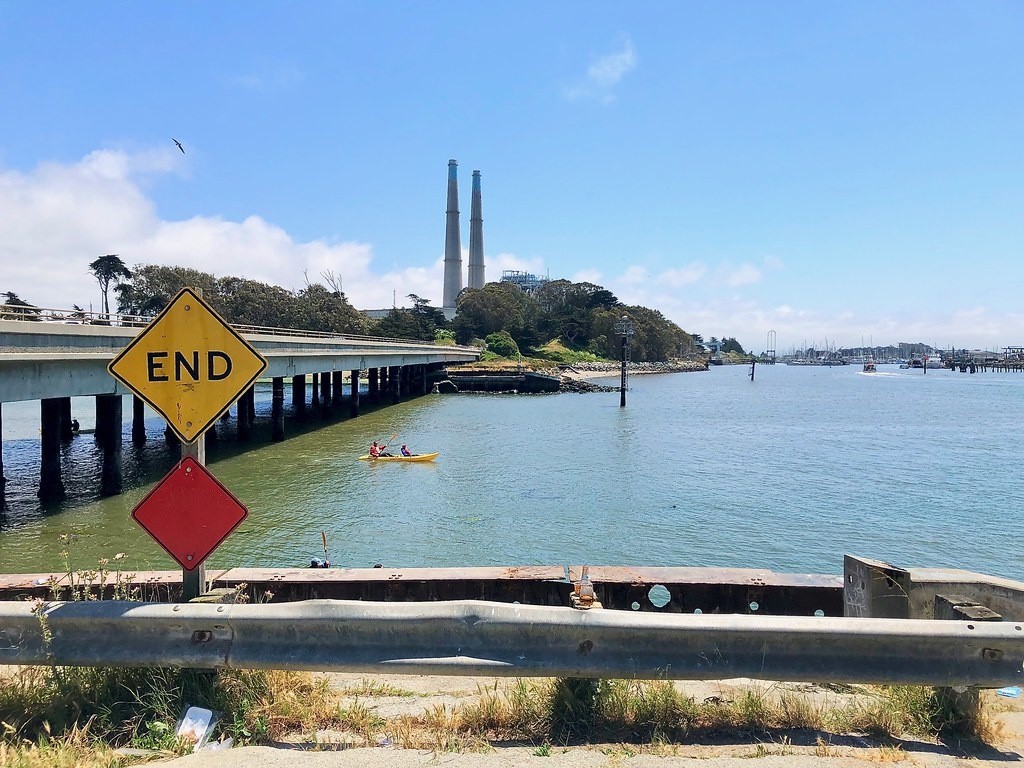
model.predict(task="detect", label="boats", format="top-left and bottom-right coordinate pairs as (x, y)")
top-left (358, 452), bottom-right (438, 462)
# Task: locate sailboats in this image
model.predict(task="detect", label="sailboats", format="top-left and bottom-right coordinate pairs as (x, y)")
top-left (785, 334), bottom-right (950, 373)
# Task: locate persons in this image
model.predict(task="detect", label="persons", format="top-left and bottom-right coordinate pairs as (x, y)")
top-left (370, 440), bottom-right (394, 457)
top-left (309, 557), bottom-right (331, 568)
top-left (71, 417), bottom-right (79, 431)
top-left (401, 443), bottom-right (419, 456)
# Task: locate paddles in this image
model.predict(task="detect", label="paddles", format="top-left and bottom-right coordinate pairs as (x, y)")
top-left (372, 434), bottom-right (397, 461)
top-left (321, 532), bottom-right (330, 568)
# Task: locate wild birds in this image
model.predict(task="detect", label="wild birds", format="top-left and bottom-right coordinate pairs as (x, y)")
top-left (171, 138), bottom-right (185, 155)
